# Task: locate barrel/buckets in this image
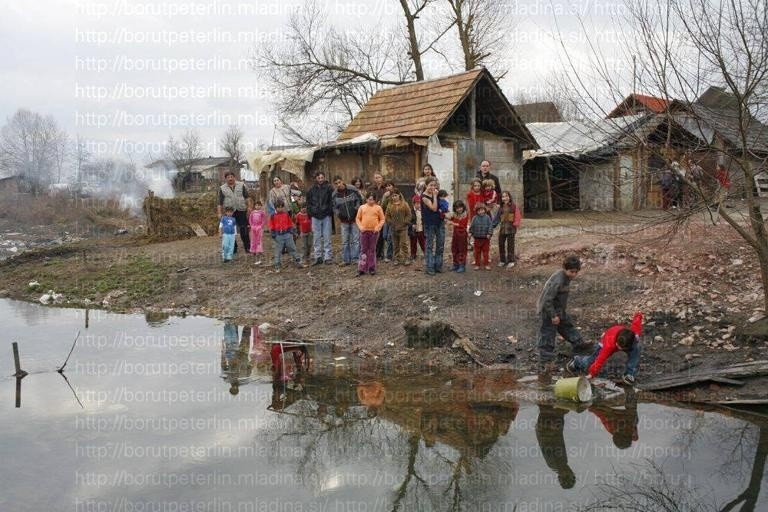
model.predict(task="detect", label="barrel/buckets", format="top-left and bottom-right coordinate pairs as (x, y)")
top-left (555, 376), bottom-right (592, 403)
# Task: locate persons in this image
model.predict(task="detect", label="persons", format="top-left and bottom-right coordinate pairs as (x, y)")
top-left (216, 160), bottom-right (523, 276)
top-left (536, 256), bottom-right (591, 378)
top-left (216, 319), bottom-right (520, 462)
top-left (668, 162), bottom-right (687, 211)
top-left (579, 396), bottom-right (652, 454)
top-left (689, 158), bottom-right (705, 209)
top-left (566, 313), bottom-right (644, 384)
top-left (713, 164), bottom-right (729, 210)
top-left (533, 398), bottom-right (582, 491)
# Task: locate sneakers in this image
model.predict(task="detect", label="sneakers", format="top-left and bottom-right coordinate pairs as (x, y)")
top-left (385, 257), bottom-right (415, 265)
top-left (566, 360), bottom-right (575, 374)
top-left (427, 264), bottom-right (465, 276)
top-left (356, 269), bottom-right (375, 276)
top-left (623, 373), bottom-right (635, 384)
top-left (275, 267), bottom-right (280, 274)
top-left (338, 263), bottom-right (351, 266)
top-left (496, 263), bottom-right (506, 267)
top-left (254, 262), bottom-right (262, 266)
top-left (583, 339), bottom-right (593, 348)
top-left (470, 259), bottom-right (492, 271)
top-left (507, 263), bottom-right (515, 268)
top-left (302, 258), bottom-right (333, 268)
